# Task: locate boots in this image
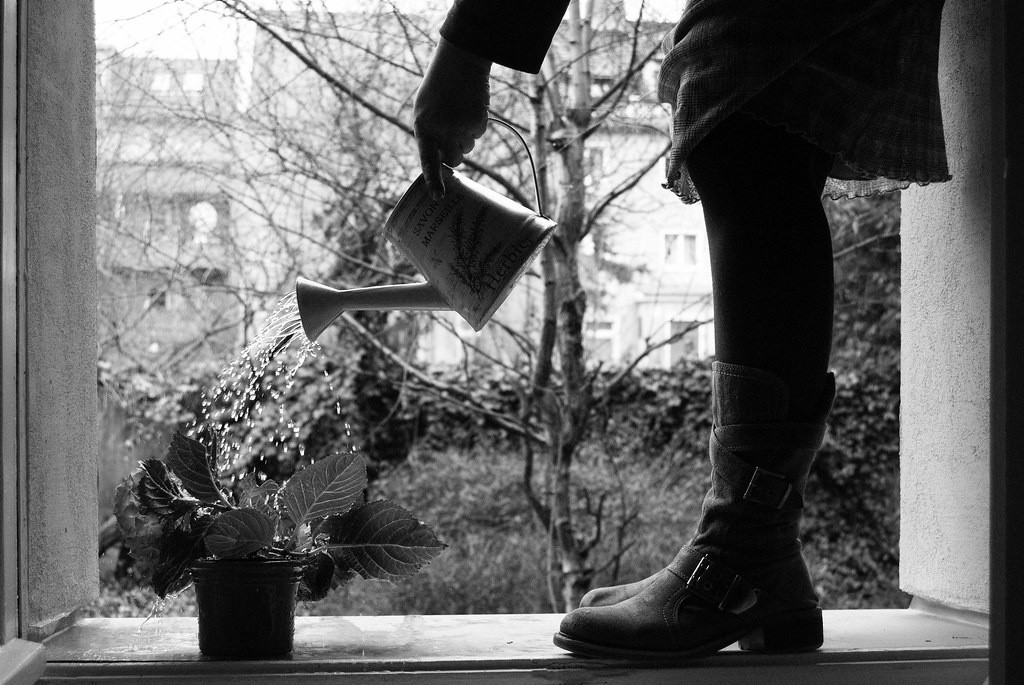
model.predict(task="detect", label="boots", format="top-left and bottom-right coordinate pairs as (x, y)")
top-left (578, 566), bottom-right (672, 609)
top-left (551, 360), bottom-right (836, 658)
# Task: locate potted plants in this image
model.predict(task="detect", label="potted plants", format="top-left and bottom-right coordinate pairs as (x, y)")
top-left (113, 426), bottom-right (449, 659)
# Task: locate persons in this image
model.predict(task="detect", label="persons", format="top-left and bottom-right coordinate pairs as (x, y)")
top-left (411, 1), bottom-right (954, 660)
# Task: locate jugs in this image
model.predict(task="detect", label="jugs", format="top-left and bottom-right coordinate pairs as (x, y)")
top-left (295, 117), bottom-right (558, 342)
